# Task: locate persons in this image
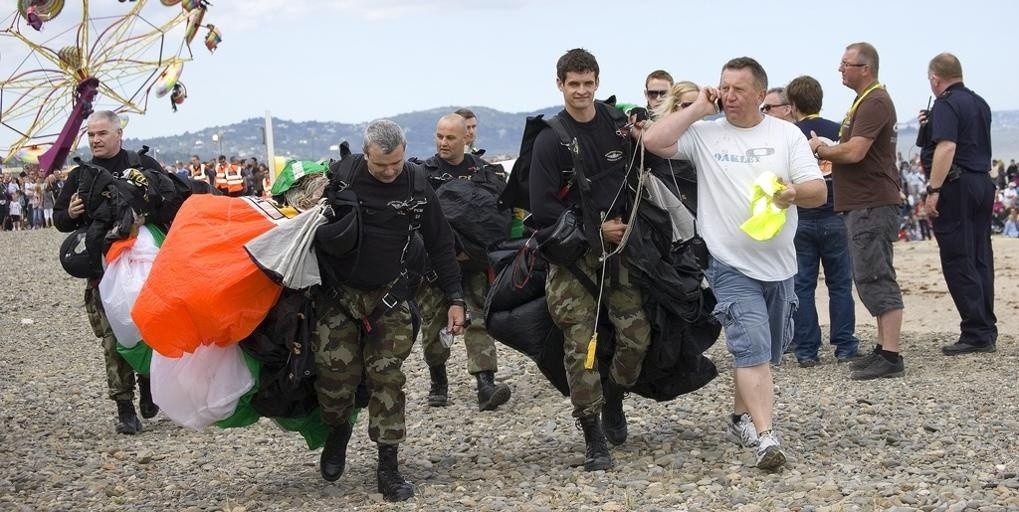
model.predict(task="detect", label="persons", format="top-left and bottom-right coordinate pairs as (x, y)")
top-left (527, 46), bottom-right (650, 474)
top-left (51, 110), bottom-right (177, 438)
top-left (407, 115), bottom-right (511, 412)
top-left (201, 21), bottom-right (221, 56)
top-left (169, 80), bottom-right (190, 112)
top-left (457, 111), bottom-right (480, 154)
top-left (28, 2), bottom-right (53, 22)
top-left (643, 54), bottom-right (829, 471)
top-left (894, 148), bottom-right (1019, 242)
top-left (914, 50), bottom-right (999, 369)
top-left (276, 119), bottom-right (467, 510)
top-left (0, 154), bottom-right (274, 233)
top-left (199, 0), bottom-right (214, 11)
top-left (808, 39), bottom-right (906, 382)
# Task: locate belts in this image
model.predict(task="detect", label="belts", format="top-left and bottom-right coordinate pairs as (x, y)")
top-left (941, 166), bottom-right (987, 183)
top-left (580, 209), bottom-right (628, 227)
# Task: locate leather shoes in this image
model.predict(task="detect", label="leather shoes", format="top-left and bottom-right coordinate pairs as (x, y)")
top-left (942, 341), bottom-right (997, 356)
top-left (850, 351), bottom-right (906, 380)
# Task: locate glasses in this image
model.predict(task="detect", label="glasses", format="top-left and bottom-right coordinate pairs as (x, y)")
top-left (676, 101), bottom-right (694, 109)
top-left (646, 90), bottom-right (669, 100)
top-left (840, 61), bottom-right (867, 68)
top-left (760, 104), bottom-right (787, 112)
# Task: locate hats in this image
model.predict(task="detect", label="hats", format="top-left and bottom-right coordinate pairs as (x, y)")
top-left (738, 171), bottom-right (789, 242)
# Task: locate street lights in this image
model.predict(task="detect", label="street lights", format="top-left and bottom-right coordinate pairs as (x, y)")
top-left (206, 134), bottom-right (224, 154)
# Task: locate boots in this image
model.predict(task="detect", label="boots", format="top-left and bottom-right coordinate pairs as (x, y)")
top-left (475, 370), bottom-right (511, 412)
top-left (376, 444), bottom-right (414, 502)
top-left (578, 412), bottom-right (612, 471)
top-left (137, 374), bottom-right (159, 419)
top-left (600, 375), bottom-right (629, 447)
top-left (318, 419), bottom-right (353, 483)
top-left (427, 366), bottom-right (450, 410)
top-left (115, 401), bottom-right (143, 434)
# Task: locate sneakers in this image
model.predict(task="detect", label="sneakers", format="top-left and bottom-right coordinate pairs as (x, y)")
top-left (754, 432), bottom-right (785, 470)
top-left (725, 412), bottom-right (758, 450)
top-left (799, 359), bottom-right (817, 367)
top-left (836, 349), bottom-right (866, 363)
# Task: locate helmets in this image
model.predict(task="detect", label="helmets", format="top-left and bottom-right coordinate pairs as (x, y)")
top-left (59, 226), bottom-right (94, 278)
top-left (313, 205), bottom-right (360, 257)
top-left (533, 209), bottom-right (589, 267)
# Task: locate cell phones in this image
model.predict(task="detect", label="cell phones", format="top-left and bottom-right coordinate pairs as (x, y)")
top-left (714, 97), bottom-right (722, 113)
